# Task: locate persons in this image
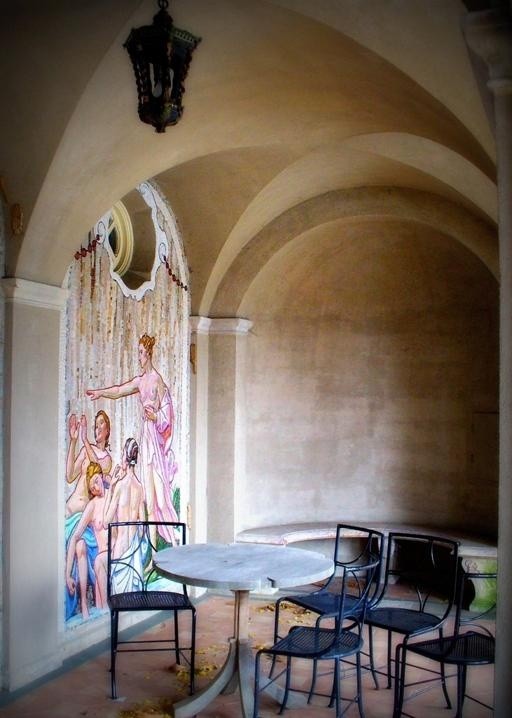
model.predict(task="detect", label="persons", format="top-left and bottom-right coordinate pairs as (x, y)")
top-left (61, 332), bottom-right (178, 617)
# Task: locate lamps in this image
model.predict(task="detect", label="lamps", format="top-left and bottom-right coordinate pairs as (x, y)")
top-left (123, 0), bottom-right (202, 132)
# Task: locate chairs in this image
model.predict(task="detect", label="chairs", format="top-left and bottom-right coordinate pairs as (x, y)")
top-left (108, 522), bottom-right (197, 699)
top-left (254, 524), bottom-right (497, 718)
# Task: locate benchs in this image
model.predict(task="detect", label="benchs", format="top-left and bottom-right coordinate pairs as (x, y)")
top-left (236, 523), bottom-right (497, 619)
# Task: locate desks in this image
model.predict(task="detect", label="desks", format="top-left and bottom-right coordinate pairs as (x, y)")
top-left (153, 544), bottom-right (335, 718)
